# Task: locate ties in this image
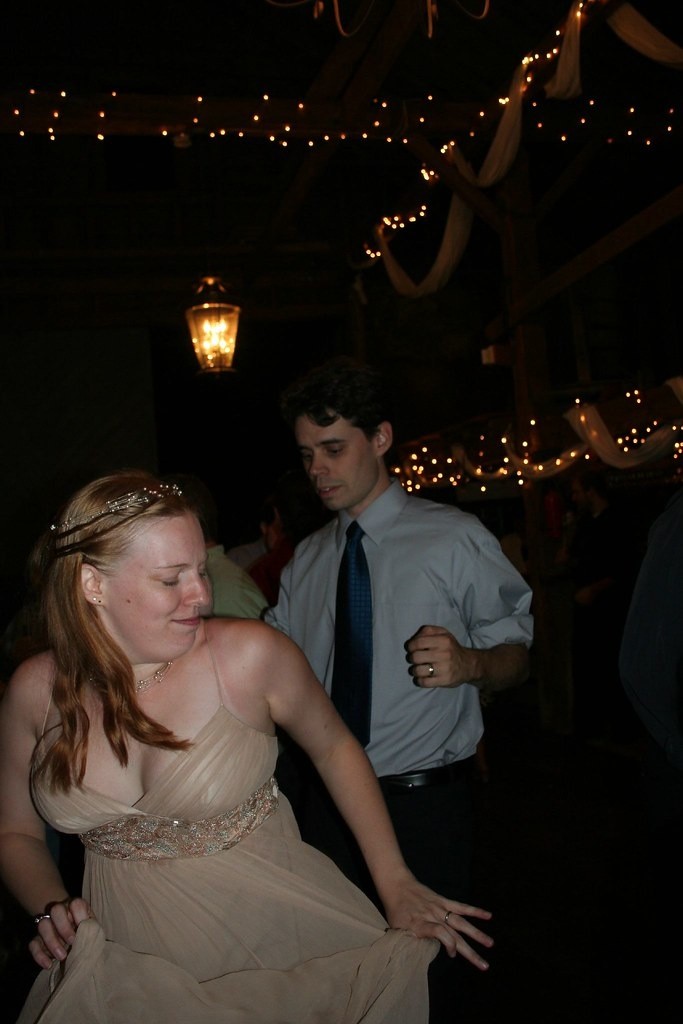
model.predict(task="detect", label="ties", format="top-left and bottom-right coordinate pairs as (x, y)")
top-left (329, 521), bottom-right (372, 748)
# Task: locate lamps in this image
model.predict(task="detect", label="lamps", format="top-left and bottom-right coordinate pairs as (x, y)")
top-left (183, 277), bottom-right (242, 382)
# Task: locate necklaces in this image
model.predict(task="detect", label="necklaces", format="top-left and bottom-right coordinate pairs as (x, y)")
top-left (89, 660), bottom-right (172, 695)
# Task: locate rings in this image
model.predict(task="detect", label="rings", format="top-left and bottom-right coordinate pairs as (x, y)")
top-left (34, 914), bottom-right (50, 925)
top-left (445, 910), bottom-right (453, 923)
top-left (428, 664), bottom-right (434, 677)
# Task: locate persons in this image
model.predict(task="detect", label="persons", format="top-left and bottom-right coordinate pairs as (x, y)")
top-left (0, 472), bottom-right (494, 1024)
top-left (263, 358), bottom-right (533, 1024)
top-left (199, 468), bottom-right (683, 768)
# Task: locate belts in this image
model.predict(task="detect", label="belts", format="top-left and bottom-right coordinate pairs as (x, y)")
top-left (378, 753), bottom-right (480, 787)
